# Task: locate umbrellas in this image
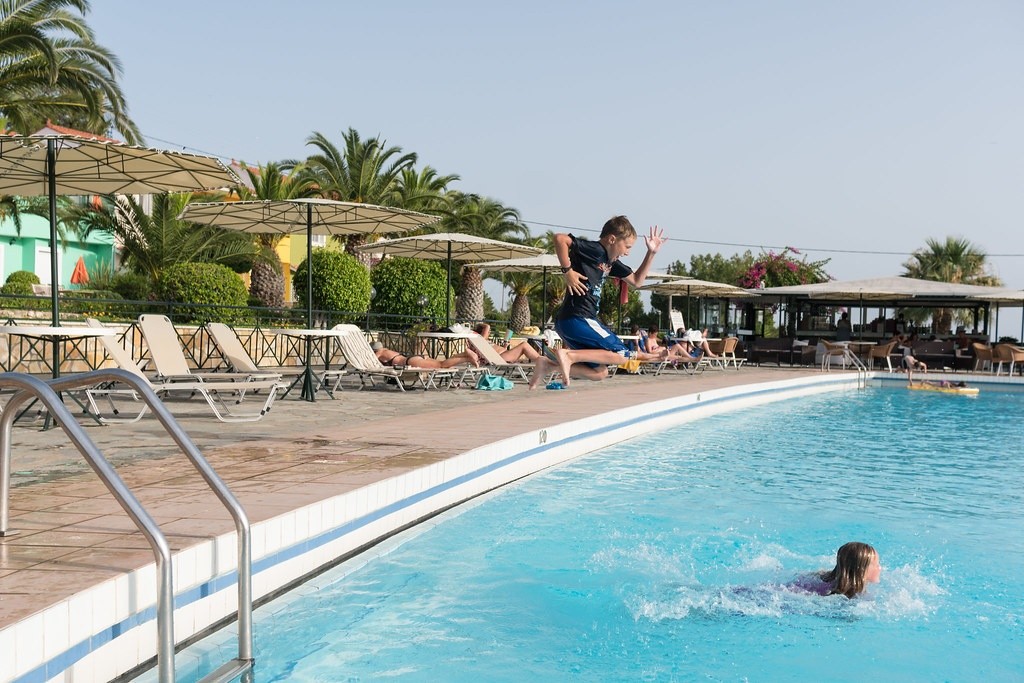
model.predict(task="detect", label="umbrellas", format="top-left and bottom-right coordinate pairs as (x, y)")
top-left (745, 275), bottom-right (1024, 369)
top-left (0, 122), bottom-right (268, 432)
top-left (175, 196), bottom-right (444, 404)
top-left (964, 290), bottom-right (1024, 350)
top-left (461, 250), bottom-right (575, 356)
top-left (605, 269), bottom-right (761, 340)
top-left (71, 256), bottom-right (90, 290)
top-left (351, 231), bottom-right (549, 391)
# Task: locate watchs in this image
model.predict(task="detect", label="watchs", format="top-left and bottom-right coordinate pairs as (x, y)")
top-left (560, 266), bottom-right (573, 274)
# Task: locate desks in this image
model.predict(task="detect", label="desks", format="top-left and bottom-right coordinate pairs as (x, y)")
top-left (843, 340), bottom-right (878, 371)
top-left (268, 327), bottom-right (349, 403)
top-left (0, 323), bottom-right (119, 430)
top-left (416, 330), bottom-right (477, 392)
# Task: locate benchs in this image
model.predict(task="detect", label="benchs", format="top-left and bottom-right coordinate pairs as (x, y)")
top-left (752, 336), bottom-right (796, 369)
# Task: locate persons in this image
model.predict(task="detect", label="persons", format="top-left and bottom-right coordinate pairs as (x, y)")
top-left (731, 542), bottom-right (882, 600)
top-left (625, 323), bottom-right (720, 368)
top-left (370, 340), bottom-right (481, 370)
top-left (836, 312), bottom-right (852, 341)
top-left (474, 322), bottom-right (544, 363)
top-left (528, 215), bottom-right (668, 392)
top-left (884, 329), bottom-right (985, 364)
top-left (920, 379), bottom-right (968, 390)
top-left (895, 355), bottom-right (928, 387)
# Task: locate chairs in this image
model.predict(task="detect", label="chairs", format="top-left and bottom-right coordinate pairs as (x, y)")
top-left (607, 335), bottom-right (748, 376)
top-left (995, 344), bottom-right (1024, 375)
top-left (820, 338), bottom-right (867, 372)
top-left (844, 340), bottom-right (873, 371)
top-left (864, 339), bottom-right (899, 372)
top-left (78, 314), bottom-right (292, 420)
top-left (329, 323), bottom-right (460, 394)
top-left (973, 343), bottom-right (992, 373)
top-left (202, 318), bottom-right (348, 403)
top-left (989, 348), bottom-right (1014, 373)
top-left (136, 314), bottom-right (282, 399)
top-left (465, 332), bottom-right (562, 388)
top-left (449, 362), bottom-right (492, 387)
